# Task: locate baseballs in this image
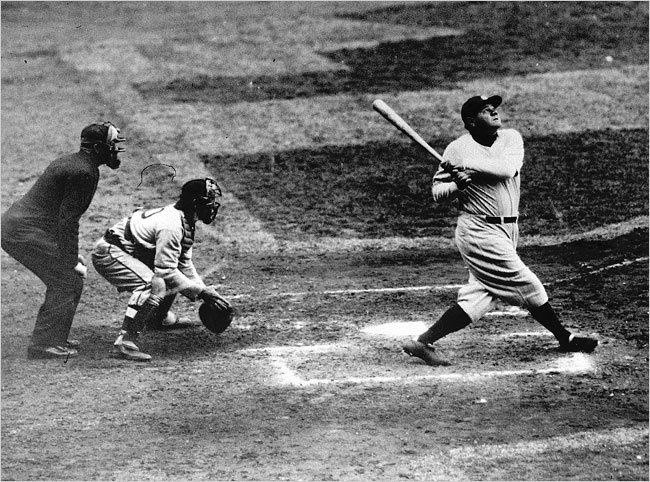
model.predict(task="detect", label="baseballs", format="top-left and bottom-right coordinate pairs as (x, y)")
top-left (604, 55), bottom-right (613, 65)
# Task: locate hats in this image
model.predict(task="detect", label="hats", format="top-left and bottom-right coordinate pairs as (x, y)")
top-left (461, 94), bottom-right (502, 116)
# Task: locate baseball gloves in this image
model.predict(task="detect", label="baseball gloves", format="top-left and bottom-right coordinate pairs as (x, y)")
top-left (198, 295), bottom-right (235, 335)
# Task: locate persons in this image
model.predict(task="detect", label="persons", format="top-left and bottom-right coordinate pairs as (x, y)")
top-left (1, 121), bottom-right (128, 360)
top-left (401, 93), bottom-right (598, 366)
top-left (91, 177), bottom-right (236, 360)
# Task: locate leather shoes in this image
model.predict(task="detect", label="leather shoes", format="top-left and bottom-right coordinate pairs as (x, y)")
top-left (27, 339), bottom-right (79, 358)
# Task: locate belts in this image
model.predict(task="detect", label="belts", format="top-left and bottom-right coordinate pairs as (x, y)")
top-left (486, 217), bottom-right (516, 224)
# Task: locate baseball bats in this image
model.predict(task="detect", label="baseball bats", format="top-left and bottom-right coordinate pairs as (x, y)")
top-left (371, 99), bottom-right (473, 186)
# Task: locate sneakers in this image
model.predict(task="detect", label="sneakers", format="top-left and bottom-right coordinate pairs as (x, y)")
top-left (560, 334), bottom-right (598, 351)
top-left (403, 339), bottom-right (451, 365)
top-left (114, 347), bottom-right (151, 361)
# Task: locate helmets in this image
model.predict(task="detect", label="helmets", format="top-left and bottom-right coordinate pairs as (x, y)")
top-left (79, 121), bottom-right (125, 169)
top-left (174, 178), bottom-right (222, 224)
